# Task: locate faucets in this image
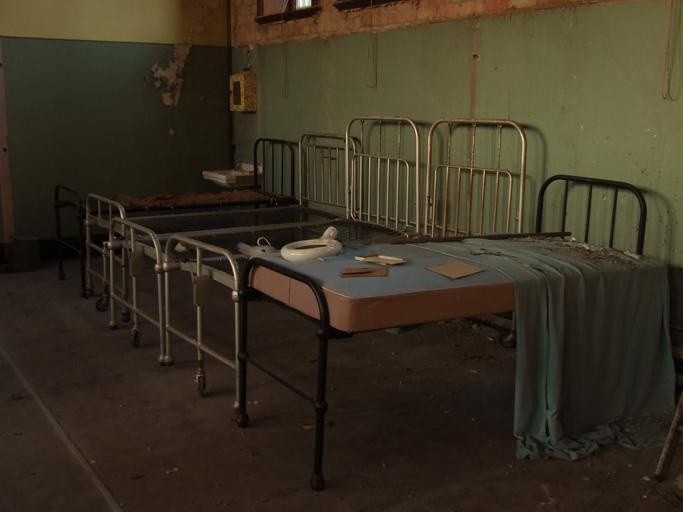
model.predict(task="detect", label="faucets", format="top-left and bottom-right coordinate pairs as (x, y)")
top-left (233, 159), bottom-right (242, 169)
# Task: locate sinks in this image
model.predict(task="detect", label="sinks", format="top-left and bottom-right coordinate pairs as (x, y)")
top-left (202, 163), bottom-right (263, 188)
